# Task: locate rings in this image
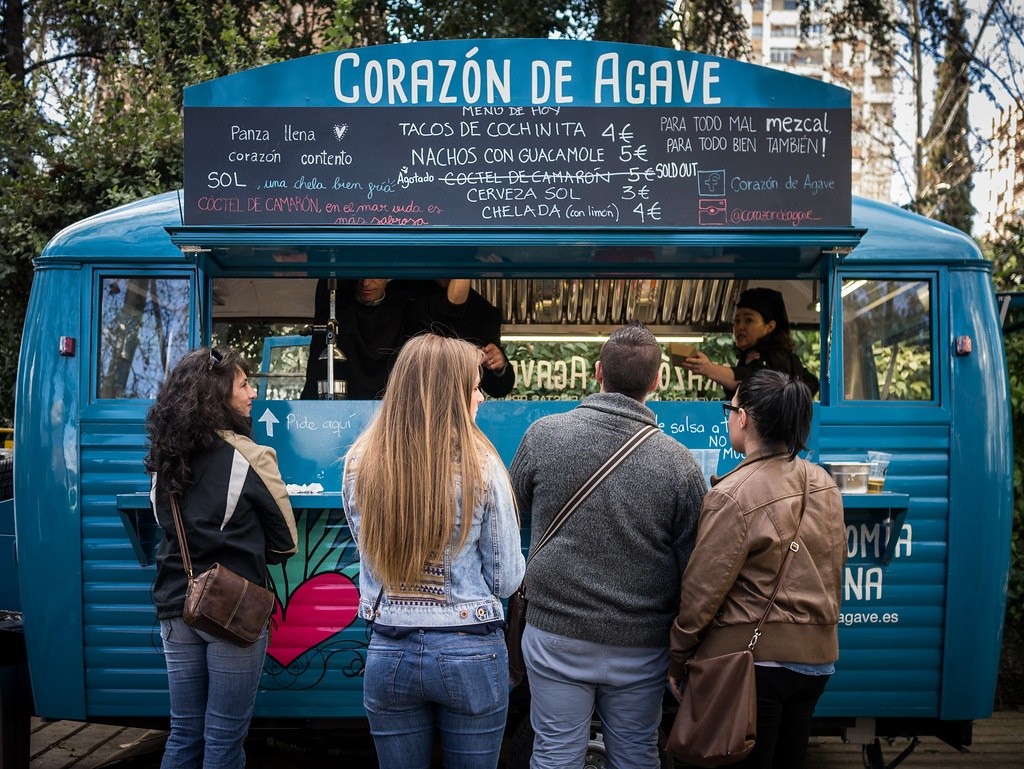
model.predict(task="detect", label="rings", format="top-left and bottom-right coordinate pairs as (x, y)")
top-left (490, 358), bottom-right (495, 364)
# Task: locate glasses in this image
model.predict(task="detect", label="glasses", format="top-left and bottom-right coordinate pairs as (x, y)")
top-left (208, 347), bottom-right (222, 369)
top-left (722, 403), bottom-right (757, 420)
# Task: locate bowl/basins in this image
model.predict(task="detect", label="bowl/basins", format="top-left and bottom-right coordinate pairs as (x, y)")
top-left (824, 460), bottom-right (871, 496)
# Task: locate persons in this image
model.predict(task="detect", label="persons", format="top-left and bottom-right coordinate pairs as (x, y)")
top-left (341, 333), bottom-right (527, 769)
top-left (683, 287), bottom-right (819, 400)
top-left (334, 277), bottom-right (516, 399)
top-left (509, 320), bottom-right (707, 769)
top-left (140, 347), bottom-right (297, 769)
top-left (668, 370), bottom-right (847, 769)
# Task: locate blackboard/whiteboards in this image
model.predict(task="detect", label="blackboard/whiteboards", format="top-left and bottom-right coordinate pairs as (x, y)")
top-left (184, 106), bottom-right (853, 228)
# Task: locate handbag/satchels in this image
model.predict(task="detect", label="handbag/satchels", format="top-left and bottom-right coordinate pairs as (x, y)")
top-left (664, 647), bottom-right (756, 757)
top-left (182, 563), bottom-right (275, 646)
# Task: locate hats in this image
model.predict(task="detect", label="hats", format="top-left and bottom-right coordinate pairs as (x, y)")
top-left (737, 287), bottom-right (789, 333)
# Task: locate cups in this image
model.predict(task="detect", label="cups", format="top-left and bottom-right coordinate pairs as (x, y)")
top-left (867, 450), bottom-right (892, 494)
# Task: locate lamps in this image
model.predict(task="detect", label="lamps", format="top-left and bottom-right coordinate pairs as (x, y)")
top-left (807, 279), bottom-right (866, 313)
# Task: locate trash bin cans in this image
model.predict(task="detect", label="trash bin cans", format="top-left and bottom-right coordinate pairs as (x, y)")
top-left (0, 610), bottom-right (31, 769)
